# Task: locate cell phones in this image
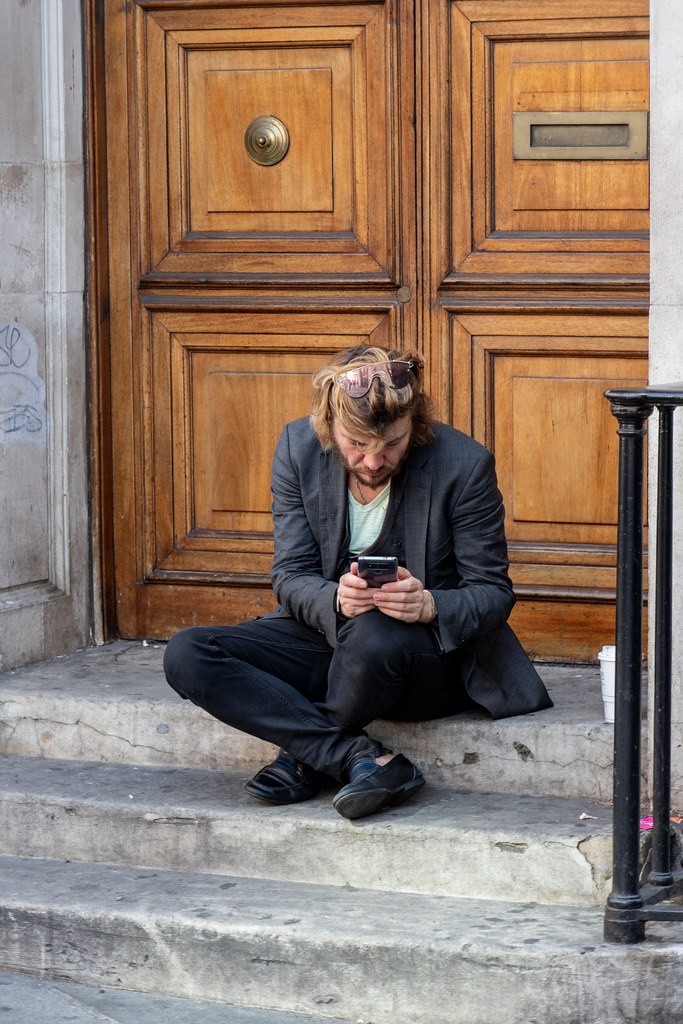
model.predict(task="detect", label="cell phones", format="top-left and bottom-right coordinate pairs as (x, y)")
top-left (357, 556), bottom-right (398, 588)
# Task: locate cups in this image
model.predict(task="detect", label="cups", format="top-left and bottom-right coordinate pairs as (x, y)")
top-left (598, 644), bottom-right (645, 724)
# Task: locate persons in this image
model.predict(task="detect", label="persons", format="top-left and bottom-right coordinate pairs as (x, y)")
top-left (163, 344), bottom-right (554, 820)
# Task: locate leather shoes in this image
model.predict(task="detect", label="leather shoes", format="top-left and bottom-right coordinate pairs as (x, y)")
top-left (333, 752), bottom-right (426, 819)
top-left (244, 753), bottom-right (327, 805)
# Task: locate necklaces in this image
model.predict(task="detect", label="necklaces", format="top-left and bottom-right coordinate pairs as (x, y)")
top-left (353, 470), bottom-right (367, 507)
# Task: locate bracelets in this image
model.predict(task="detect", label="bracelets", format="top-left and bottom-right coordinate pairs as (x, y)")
top-left (423, 589), bottom-right (437, 624)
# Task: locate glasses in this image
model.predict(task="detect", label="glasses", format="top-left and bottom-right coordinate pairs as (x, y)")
top-left (336, 361), bottom-right (413, 398)
